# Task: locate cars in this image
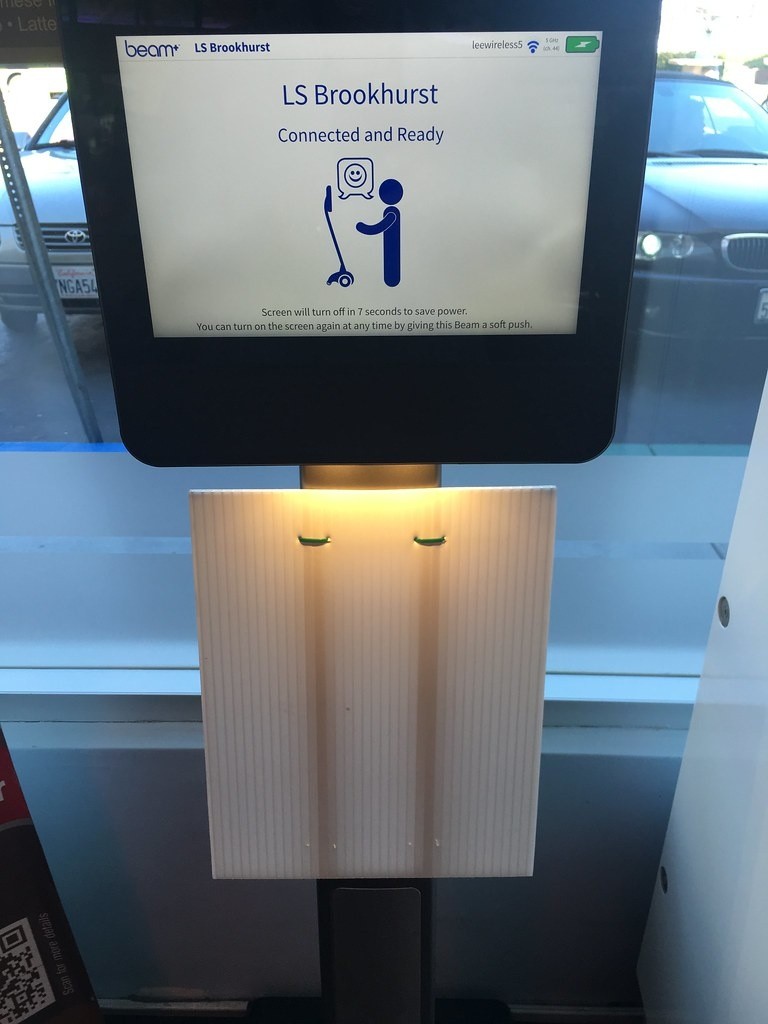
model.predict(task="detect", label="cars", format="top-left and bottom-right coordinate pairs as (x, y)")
top-left (0, 66), bottom-right (103, 330)
top-left (622, 66), bottom-right (768, 399)
